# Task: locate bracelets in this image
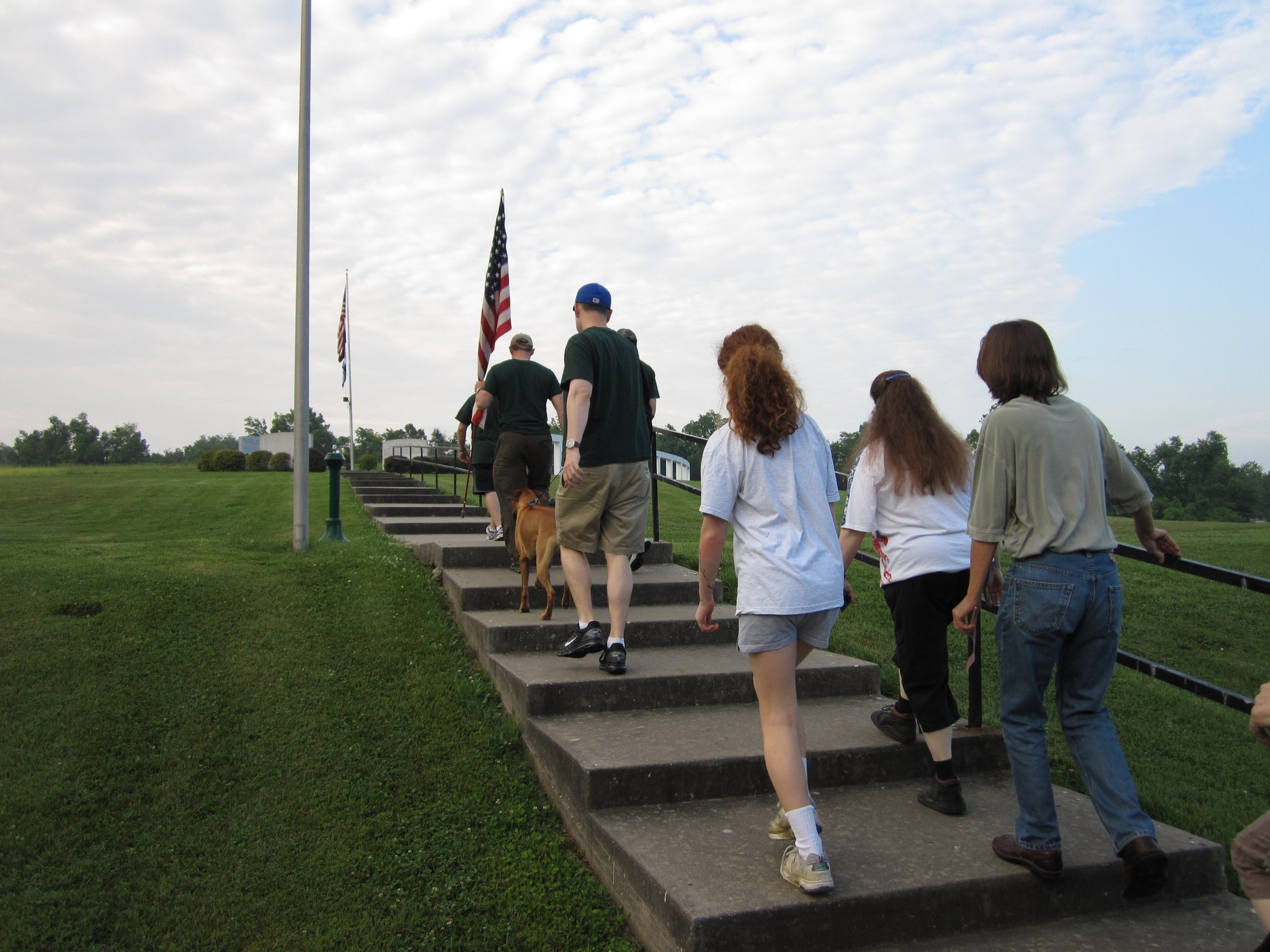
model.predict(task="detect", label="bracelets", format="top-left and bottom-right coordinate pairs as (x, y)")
top-left (458, 447), bottom-right (466, 452)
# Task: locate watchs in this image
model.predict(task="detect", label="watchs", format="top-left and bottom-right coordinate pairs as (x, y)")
top-left (565, 439), bottom-right (580, 449)
top-left (476, 387), bottom-right (483, 394)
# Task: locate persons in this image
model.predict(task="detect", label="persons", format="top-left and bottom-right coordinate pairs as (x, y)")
top-left (616, 328), bottom-right (660, 572)
top-left (838, 370), bottom-right (1005, 815)
top-left (555, 287), bottom-right (653, 675)
top-left (695, 326), bottom-right (855, 895)
top-left (474, 333), bottom-right (564, 574)
top-left (455, 393), bottom-right (512, 541)
top-left (1230, 682), bottom-right (1270, 952)
top-left (952, 324), bottom-right (1184, 904)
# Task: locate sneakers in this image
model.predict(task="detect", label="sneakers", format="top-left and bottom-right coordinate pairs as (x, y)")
top-left (494, 527), bottom-right (504, 540)
top-left (768, 796), bottom-right (823, 840)
top-left (598, 642), bottom-right (627, 675)
top-left (871, 704), bottom-right (916, 744)
top-left (779, 840), bottom-right (835, 895)
top-left (486, 524), bottom-right (497, 542)
top-left (557, 622), bottom-right (604, 660)
top-left (916, 772), bottom-right (967, 815)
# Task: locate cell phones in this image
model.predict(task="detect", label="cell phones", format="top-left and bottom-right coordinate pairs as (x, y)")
top-left (970, 606), bottom-right (977, 624)
top-left (841, 590), bottom-right (851, 613)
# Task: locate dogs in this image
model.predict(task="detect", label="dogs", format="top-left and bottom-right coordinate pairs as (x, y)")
top-left (510, 487), bottom-right (571, 619)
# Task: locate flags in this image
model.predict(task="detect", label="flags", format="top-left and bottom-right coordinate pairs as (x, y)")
top-left (471, 195), bottom-right (512, 431)
top-left (336, 286), bottom-right (347, 388)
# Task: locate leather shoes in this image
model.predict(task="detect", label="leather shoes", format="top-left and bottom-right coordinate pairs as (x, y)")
top-left (1123, 837), bottom-right (1168, 895)
top-left (991, 832), bottom-right (1064, 879)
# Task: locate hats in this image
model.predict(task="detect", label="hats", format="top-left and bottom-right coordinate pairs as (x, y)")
top-left (571, 282), bottom-right (612, 311)
top-left (618, 329), bottom-right (637, 343)
top-left (510, 333), bottom-right (534, 347)
